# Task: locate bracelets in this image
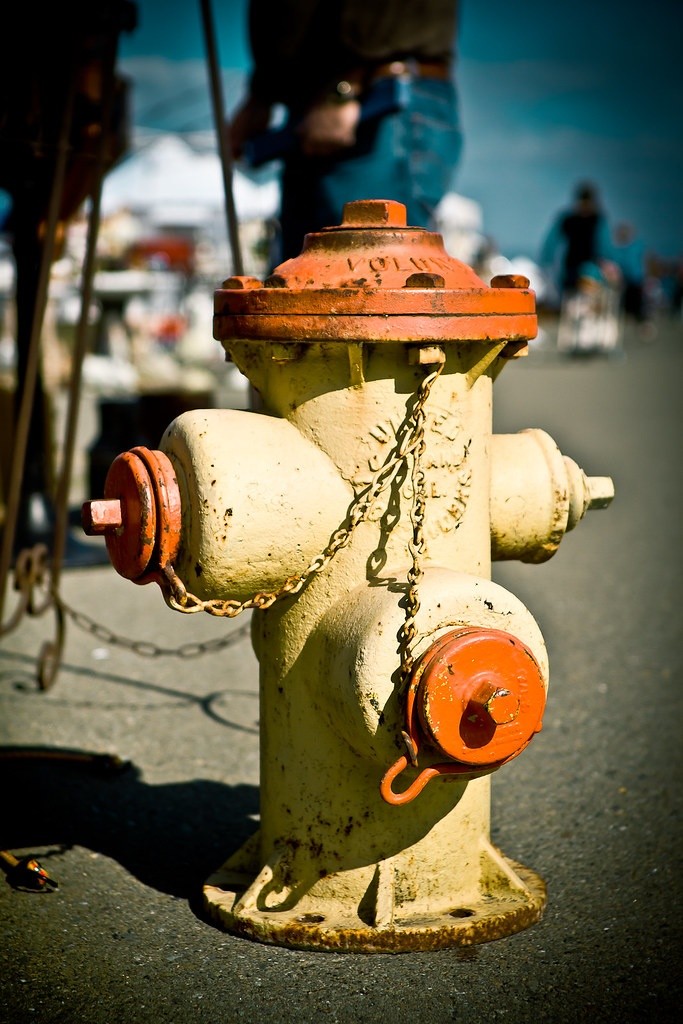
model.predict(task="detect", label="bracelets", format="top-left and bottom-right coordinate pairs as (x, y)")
top-left (332, 82), bottom-right (353, 101)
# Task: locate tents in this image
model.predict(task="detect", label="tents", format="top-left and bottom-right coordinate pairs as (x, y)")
top-left (84, 136), bottom-right (280, 230)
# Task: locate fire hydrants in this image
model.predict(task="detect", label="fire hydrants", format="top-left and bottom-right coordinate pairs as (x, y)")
top-left (96, 208), bottom-right (620, 954)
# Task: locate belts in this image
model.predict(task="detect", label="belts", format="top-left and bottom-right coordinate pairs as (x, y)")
top-left (373, 61), bottom-right (449, 79)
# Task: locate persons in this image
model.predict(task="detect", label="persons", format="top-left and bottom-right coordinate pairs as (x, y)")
top-left (559, 180), bottom-right (651, 324)
top-left (225, 0), bottom-right (464, 261)
